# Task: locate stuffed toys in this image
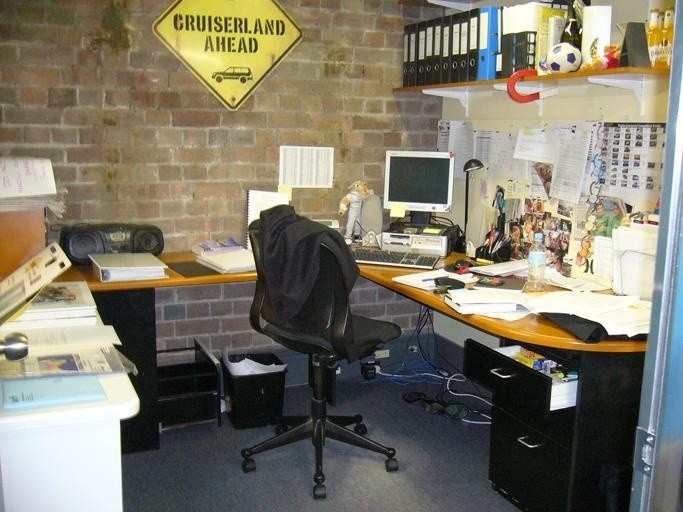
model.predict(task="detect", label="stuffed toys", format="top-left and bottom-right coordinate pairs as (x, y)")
top-left (337, 179), bottom-right (373, 246)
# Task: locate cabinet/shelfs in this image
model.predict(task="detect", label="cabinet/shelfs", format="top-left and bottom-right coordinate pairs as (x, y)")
top-left (0, 281), bottom-right (140, 512)
top-left (0, 205), bottom-right (48, 281)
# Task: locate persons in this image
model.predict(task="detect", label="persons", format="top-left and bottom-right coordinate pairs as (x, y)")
top-left (607, 202), bottom-right (621, 238)
top-left (508, 199), bottom-right (571, 263)
top-left (589, 202), bottom-right (612, 237)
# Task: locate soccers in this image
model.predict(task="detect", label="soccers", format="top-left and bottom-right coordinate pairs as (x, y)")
top-left (547, 42), bottom-right (581, 73)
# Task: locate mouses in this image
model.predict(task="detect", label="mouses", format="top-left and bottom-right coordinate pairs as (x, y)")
top-left (454, 260), bottom-right (473, 271)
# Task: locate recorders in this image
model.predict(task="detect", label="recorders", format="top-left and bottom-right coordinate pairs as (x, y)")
top-left (58, 222), bottom-right (164, 265)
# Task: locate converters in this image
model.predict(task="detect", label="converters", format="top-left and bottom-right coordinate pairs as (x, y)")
top-left (363, 364), bottom-right (376, 379)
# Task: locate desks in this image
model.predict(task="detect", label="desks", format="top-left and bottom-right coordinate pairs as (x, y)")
top-left (53, 247), bottom-right (650, 512)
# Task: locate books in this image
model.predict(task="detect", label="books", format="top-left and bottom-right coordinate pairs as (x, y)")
top-left (191, 236), bottom-right (256, 275)
top-left (445, 294), bottom-right (517, 315)
top-left (0, 156), bottom-right (123, 414)
top-left (246, 188), bottom-right (289, 252)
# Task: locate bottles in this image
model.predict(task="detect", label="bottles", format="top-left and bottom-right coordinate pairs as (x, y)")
top-left (560, 0), bottom-right (581, 52)
top-left (527, 233), bottom-right (547, 292)
top-left (646, 5), bottom-right (675, 69)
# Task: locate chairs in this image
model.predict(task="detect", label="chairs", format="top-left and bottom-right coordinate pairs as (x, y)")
top-left (241, 218), bottom-right (402, 501)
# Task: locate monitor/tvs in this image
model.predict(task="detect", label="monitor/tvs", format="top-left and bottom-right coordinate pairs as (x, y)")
top-left (382, 149), bottom-right (456, 233)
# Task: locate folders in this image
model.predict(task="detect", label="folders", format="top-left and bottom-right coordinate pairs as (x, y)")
top-left (403, 6), bottom-right (498, 87)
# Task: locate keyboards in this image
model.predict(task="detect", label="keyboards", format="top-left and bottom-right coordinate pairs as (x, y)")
top-left (350, 247), bottom-right (441, 270)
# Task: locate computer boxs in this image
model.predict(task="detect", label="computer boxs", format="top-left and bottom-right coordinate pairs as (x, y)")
top-left (381, 222), bottom-right (460, 257)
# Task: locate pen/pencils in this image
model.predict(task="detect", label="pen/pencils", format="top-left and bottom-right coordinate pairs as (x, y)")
top-left (476, 257), bottom-right (495, 265)
top-left (480, 224), bottom-right (512, 255)
top-left (423, 276), bottom-right (449, 282)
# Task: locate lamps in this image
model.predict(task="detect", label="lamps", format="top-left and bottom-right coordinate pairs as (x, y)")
top-left (451, 158), bottom-right (484, 253)
